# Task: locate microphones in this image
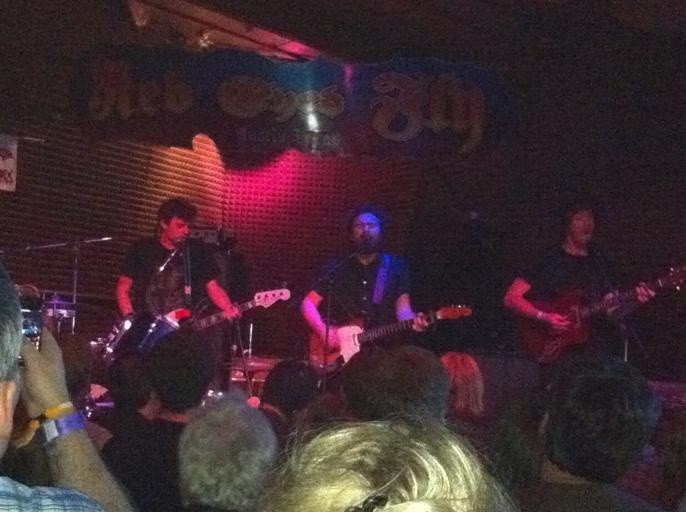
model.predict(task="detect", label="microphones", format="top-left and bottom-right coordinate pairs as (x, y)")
top-left (585, 232), bottom-right (600, 254)
top-left (361, 231), bottom-right (373, 246)
top-left (173, 237), bottom-right (183, 249)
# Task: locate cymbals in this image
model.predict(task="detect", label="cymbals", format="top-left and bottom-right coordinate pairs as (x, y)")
top-left (43, 298), bottom-right (74, 305)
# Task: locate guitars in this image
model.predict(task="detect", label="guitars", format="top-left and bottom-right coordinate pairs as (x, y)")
top-left (310, 305), bottom-right (472, 376)
top-left (516, 266), bottom-right (685, 363)
top-left (163, 285), bottom-right (291, 331)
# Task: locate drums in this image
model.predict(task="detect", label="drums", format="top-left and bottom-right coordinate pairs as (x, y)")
top-left (103, 309), bottom-right (181, 360)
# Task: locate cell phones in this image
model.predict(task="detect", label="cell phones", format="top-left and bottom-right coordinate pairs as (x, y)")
top-left (15, 296), bottom-right (45, 367)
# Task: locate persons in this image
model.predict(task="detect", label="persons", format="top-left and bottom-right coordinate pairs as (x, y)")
top-left (298, 205), bottom-right (431, 372)
top-left (504, 201), bottom-right (658, 378)
top-left (115, 191), bottom-right (241, 407)
top-left (1, 269), bottom-right (130, 512)
top-left (25, 324), bottom-right (686, 511)
